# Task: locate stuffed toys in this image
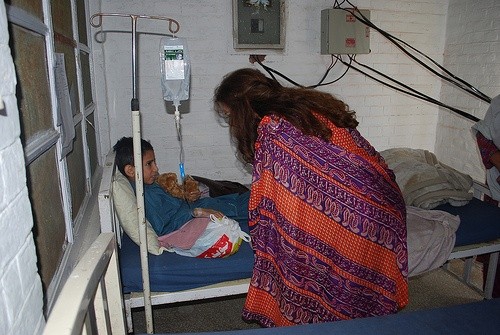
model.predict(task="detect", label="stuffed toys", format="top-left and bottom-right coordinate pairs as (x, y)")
top-left (155, 172), bottom-right (200, 202)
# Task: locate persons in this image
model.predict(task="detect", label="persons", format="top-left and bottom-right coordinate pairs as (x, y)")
top-left (213, 69), bottom-right (409, 329)
top-left (472, 94), bottom-right (500, 203)
top-left (114, 135), bottom-right (252, 239)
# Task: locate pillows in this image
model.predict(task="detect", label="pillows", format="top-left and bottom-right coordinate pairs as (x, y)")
top-left (112, 169), bottom-right (164, 255)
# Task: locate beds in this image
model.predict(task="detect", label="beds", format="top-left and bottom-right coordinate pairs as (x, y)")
top-left (98, 149), bottom-right (500, 331)
top-left (41, 230), bottom-right (500, 335)
